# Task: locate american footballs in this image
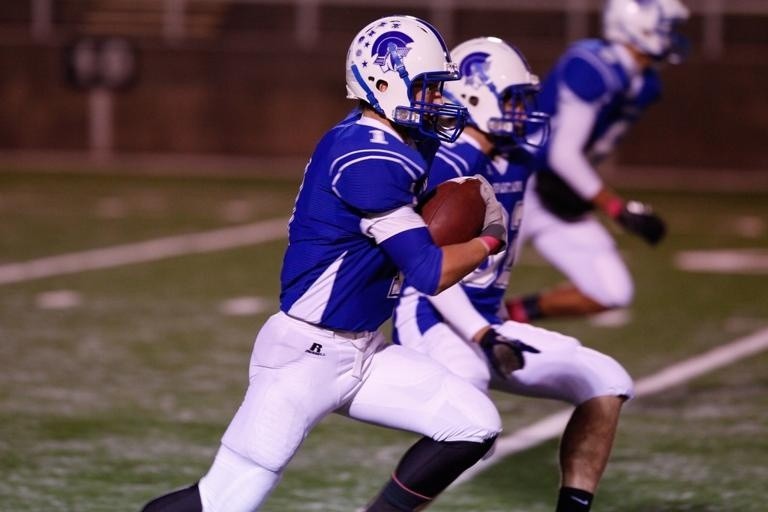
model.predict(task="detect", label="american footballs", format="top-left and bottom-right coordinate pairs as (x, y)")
top-left (415, 177), bottom-right (486, 247)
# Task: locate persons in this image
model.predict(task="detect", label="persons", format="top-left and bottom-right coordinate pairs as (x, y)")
top-left (138, 13), bottom-right (509, 510)
top-left (396, 33), bottom-right (637, 512)
top-left (499, 0), bottom-right (694, 325)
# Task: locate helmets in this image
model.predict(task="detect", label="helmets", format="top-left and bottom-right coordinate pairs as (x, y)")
top-left (436, 34), bottom-right (551, 159)
top-left (600, 1), bottom-right (693, 70)
top-left (343, 13), bottom-right (469, 145)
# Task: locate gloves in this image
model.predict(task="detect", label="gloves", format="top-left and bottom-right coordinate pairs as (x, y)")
top-left (477, 327), bottom-right (542, 378)
top-left (613, 201), bottom-right (664, 246)
top-left (475, 172), bottom-right (511, 255)
